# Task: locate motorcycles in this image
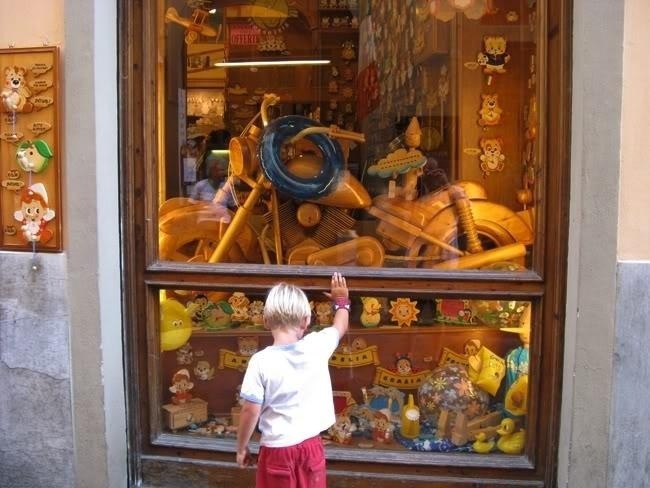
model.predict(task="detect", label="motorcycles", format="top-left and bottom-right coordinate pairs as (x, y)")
top-left (158, 92), bottom-right (535, 272)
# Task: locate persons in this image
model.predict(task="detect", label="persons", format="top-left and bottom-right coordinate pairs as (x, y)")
top-left (189, 151), bottom-right (251, 235)
top-left (235, 272), bottom-right (351, 488)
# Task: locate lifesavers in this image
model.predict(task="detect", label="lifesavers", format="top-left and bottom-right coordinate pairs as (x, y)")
top-left (258, 114), bottom-right (345, 200)
